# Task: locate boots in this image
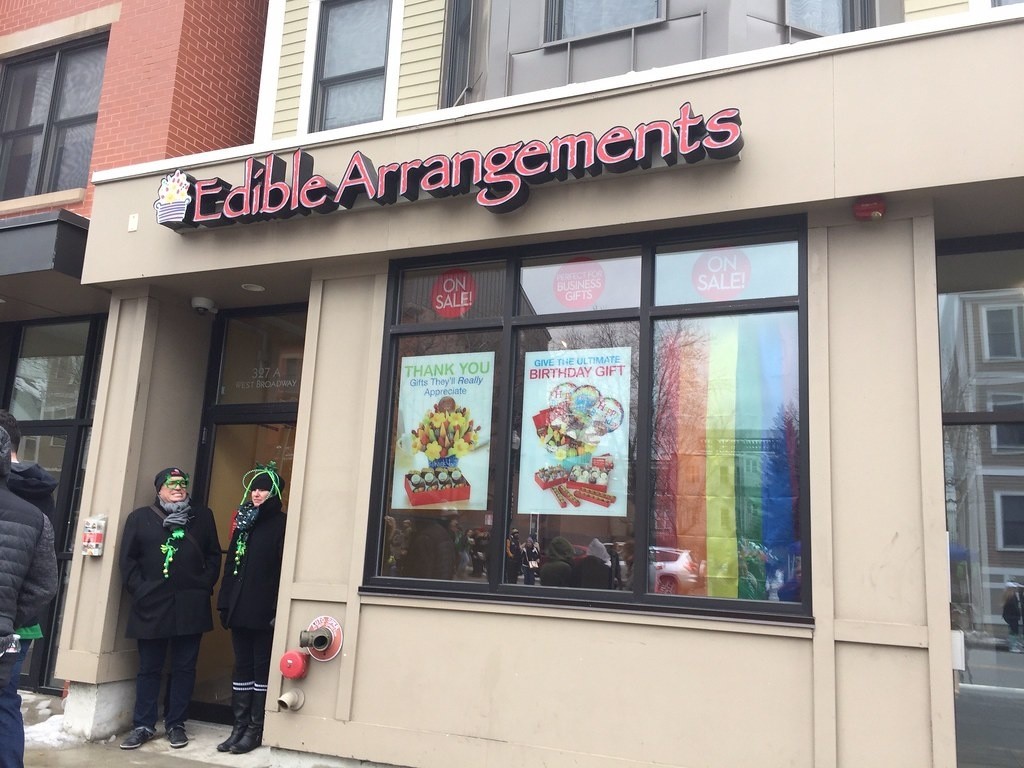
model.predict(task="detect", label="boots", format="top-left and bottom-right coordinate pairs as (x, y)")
top-left (230, 680), bottom-right (267, 754)
top-left (217, 679), bottom-right (255, 752)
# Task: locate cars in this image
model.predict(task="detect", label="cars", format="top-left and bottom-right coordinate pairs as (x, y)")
top-left (539, 544), bottom-right (590, 565)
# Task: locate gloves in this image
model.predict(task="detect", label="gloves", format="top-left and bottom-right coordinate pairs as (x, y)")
top-left (220, 609), bottom-right (229, 630)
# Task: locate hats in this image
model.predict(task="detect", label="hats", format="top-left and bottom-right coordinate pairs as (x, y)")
top-left (249, 473), bottom-right (285, 495)
top-left (155, 467), bottom-right (189, 495)
top-left (510, 527), bottom-right (519, 535)
top-left (526, 537), bottom-right (534, 544)
top-left (403, 519), bottom-right (411, 523)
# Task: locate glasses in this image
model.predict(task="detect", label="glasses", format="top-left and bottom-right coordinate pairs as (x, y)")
top-left (163, 474), bottom-right (189, 488)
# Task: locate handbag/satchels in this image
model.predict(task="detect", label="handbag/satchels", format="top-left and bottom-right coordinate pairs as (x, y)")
top-left (478, 551), bottom-right (486, 560)
top-left (528, 561), bottom-right (539, 568)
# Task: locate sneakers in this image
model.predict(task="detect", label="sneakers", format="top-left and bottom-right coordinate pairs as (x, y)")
top-left (166, 724), bottom-right (188, 748)
top-left (120, 726), bottom-right (156, 748)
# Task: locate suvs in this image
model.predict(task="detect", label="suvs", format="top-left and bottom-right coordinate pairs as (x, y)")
top-left (605, 546), bottom-right (706, 595)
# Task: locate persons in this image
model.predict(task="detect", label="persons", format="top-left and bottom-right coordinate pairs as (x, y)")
top-left (506, 529), bottom-right (634, 590)
top-left (1001, 582), bottom-right (1024, 653)
top-left (736, 537), bottom-right (802, 603)
top-left (383, 510), bottom-right (487, 579)
top-left (119, 468), bottom-right (222, 749)
top-left (0, 409), bottom-right (58, 768)
top-left (218, 462), bottom-right (288, 753)
